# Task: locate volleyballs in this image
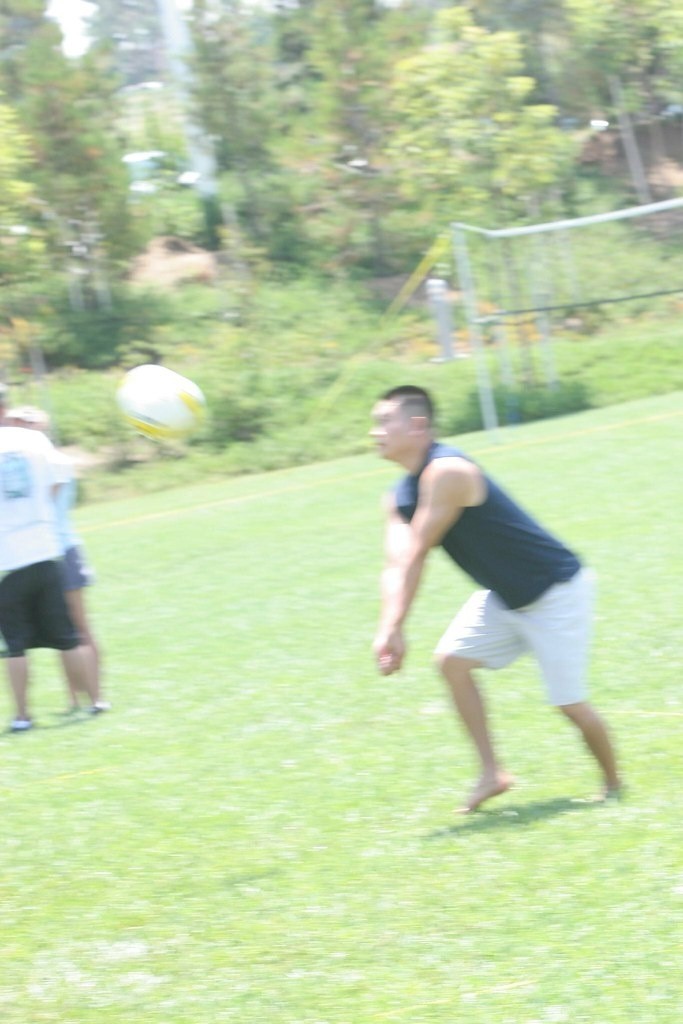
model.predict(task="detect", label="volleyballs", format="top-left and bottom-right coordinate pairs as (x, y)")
top-left (115, 361), bottom-right (211, 446)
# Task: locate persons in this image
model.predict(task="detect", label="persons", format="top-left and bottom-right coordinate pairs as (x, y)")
top-left (0, 387), bottom-right (110, 728)
top-left (5, 405), bottom-right (105, 716)
top-left (368, 385), bottom-right (628, 812)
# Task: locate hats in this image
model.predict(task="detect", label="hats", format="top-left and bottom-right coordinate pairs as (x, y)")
top-left (4, 407), bottom-right (48, 423)
